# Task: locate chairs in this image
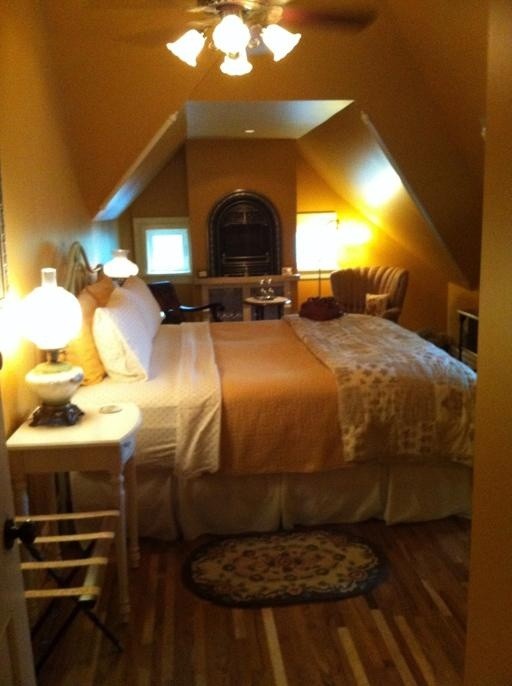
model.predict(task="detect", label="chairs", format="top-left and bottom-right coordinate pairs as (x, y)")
top-left (148, 277), bottom-right (228, 327)
top-left (327, 261), bottom-right (411, 326)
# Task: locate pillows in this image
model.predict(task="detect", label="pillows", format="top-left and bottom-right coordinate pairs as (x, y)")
top-left (365, 292), bottom-right (392, 319)
top-left (121, 270), bottom-right (165, 337)
top-left (92, 286), bottom-right (159, 382)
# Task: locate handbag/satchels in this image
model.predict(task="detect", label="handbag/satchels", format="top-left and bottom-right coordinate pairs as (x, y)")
top-left (299, 296), bottom-right (345, 321)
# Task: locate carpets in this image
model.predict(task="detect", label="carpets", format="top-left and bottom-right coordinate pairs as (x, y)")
top-left (178, 526), bottom-right (389, 613)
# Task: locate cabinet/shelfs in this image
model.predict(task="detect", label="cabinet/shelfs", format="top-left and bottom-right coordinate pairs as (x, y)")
top-left (195, 271), bottom-right (300, 322)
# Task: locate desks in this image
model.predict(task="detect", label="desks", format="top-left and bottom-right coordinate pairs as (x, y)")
top-left (241, 294), bottom-right (294, 320)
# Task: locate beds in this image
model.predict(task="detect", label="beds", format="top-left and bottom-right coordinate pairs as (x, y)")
top-left (45, 236), bottom-right (476, 549)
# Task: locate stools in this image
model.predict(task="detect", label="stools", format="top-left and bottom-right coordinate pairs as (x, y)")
top-left (6, 506), bottom-right (124, 670)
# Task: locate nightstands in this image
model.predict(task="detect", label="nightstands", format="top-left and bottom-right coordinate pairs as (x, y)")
top-left (4, 398), bottom-right (152, 626)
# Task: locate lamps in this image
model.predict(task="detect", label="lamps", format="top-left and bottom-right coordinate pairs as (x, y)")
top-left (100, 247), bottom-right (140, 283)
top-left (162, 0), bottom-right (304, 77)
top-left (23, 265), bottom-right (86, 428)
